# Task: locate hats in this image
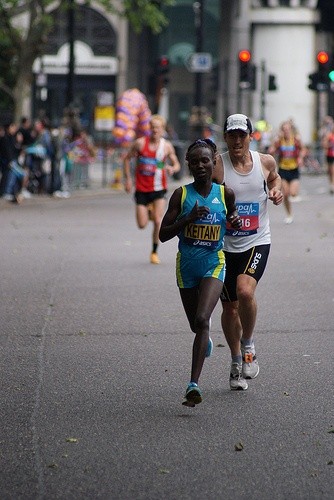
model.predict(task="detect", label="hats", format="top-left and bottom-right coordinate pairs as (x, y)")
top-left (224, 113), bottom-right (252, 134)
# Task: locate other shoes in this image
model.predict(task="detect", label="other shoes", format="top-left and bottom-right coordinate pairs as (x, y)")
top-left (150, 252), bottom-right (160, 264)
top-left (147, 203), bottom-right (155, 221)
top-left (285, 217), bottom-right (293, 224)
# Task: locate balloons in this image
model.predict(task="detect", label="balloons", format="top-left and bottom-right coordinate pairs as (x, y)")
top-left (113, 88), bottom-right (151, 146)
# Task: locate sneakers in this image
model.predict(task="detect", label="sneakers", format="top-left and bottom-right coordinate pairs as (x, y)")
top-left (206, 317), bottom-right (213, 357)
top-left (240, 340), bottom-right (259, 378)
top-left (182, 381), bottom-right (202, 408)
top-left (229, 362), bottom-right (248, 391)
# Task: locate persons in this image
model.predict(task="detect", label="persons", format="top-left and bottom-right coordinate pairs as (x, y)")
top-left (160, 138), bottom-right (242, 406)
top-left (266, 121), bottom-right (307, 224)
top-left (322, 121), bottom-right (334, 197)
top-left (49, 124), bottom-right (97, 197)
top-left (123, 115), bottom-right (181, 264)
top-left (212, 114), bottom-right (283, 390)
top-left (0, 117), bottom-right (62, 201)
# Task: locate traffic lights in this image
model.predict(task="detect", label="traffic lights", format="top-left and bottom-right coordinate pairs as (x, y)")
top-left (147, 73), bottom-right (159, 94)
top-left (159, 56), bottom-right (170, 85)
top-left (238, 50), bottom-right (252, 80)
top-left (269, 74), bottom-right (276, 89)
top-left (309, 72), bottom-right (318, 91)
top-left (316, 50), bottom-right (329, 83)
